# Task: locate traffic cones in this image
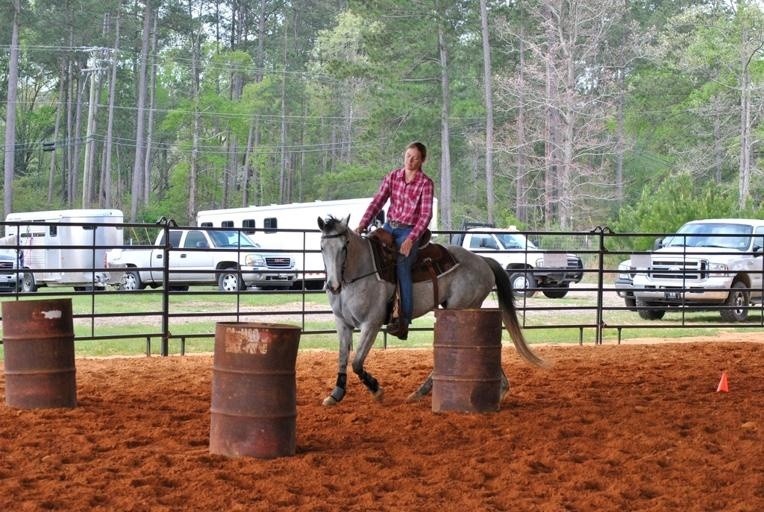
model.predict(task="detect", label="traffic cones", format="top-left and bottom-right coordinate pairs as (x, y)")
top-left (717, 373), bottom-right (728, 393)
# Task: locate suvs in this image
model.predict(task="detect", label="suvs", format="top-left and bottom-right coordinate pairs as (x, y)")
top-left (614, 218), bottom-right (764, 322)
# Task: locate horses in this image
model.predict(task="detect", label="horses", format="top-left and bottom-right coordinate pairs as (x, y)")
top-left (316, 213), bottom-right (544, 408)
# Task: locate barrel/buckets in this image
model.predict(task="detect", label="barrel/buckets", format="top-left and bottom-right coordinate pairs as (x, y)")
top-left (0, 299), bottom-right (78, 408)
top-left (208, 320), bottom-right (300, 461)
top-left (430, 307), bottom-right (503, 415)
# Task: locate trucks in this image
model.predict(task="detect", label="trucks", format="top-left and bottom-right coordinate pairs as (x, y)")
top-left (4, 208), bottom-right (124, 292)
top-left (196, 197), bottom-right (583, 298)
top-left (0, 249), bottom-right (25, 292)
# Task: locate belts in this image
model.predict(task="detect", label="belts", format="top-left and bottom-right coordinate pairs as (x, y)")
top-left (388, 219), bottom-right (411, 229)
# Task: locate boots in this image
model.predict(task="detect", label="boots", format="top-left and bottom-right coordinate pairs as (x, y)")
top-left (387, 318), bottom-right (410, 340)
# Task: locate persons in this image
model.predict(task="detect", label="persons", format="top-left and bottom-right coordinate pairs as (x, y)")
top-left (353, 140), bottom-right (435, 340)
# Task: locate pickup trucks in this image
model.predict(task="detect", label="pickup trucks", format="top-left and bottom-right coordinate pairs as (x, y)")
top-left (105, 229), bottom-right (298, 291)
